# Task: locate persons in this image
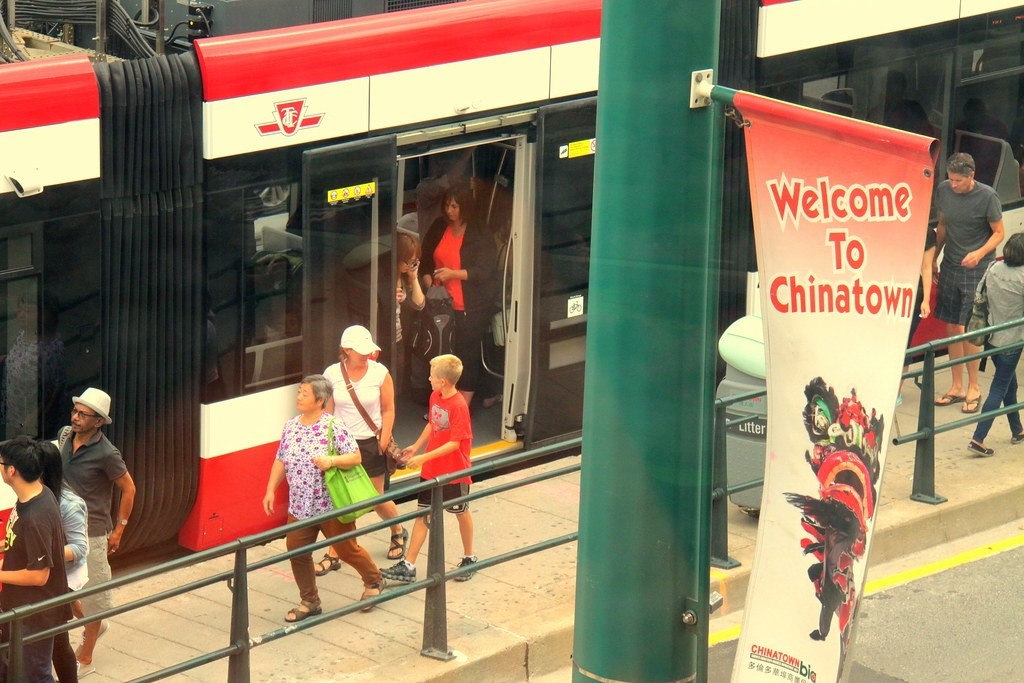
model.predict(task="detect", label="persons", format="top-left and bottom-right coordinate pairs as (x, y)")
top-left (417, 183), bottom-right (504, 420)
top-left (929, 152), bottom-right (1005, 413)
top-left (968, 232), bottom-right (1024, 456)
top-left (896, 225), bottom-right (936, 406)
top-left (263, 373), bottom-right (388, 622)
top-left (0, 387), bottom-right (136, 683)
top-left (380, 355), bottom-right (479, 583)
top-left (311, 324), bottom-right (409, 576)
top-left (393, 232), bottom-right (425, 397)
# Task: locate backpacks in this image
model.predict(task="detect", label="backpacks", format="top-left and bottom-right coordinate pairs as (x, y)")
top-left (410, 280), bottom-right (456, 361)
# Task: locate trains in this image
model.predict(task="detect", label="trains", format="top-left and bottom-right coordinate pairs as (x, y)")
top-left (0, 0), bottom-right (1024, 565)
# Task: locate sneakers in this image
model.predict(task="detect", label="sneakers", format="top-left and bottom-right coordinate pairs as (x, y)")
top-left (379, 561), bottom-right (418, 582)
top-left (455, 557), bottom-right (478, 581)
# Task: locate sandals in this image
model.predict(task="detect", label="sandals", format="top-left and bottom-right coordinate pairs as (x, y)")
top-left (360, 577), bottom-right (386, 611)
top-left (388, 526), bottom-right (408, 560)
top-left (284, 599), bottom-right (321, 623)
top-left (313, 552), bottom-right (340, 575)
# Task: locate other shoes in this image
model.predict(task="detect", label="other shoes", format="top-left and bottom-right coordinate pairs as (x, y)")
top-left (1012, 432), bottom-right (1024, 444)
top-left (967, 440), bottom-right (994, 456)
top-left (483, 393), bottom-right (504, 408)
top-left (56, 658), bottom-right (93, 681)
top-left (74, 620), bottom-right (109, 658)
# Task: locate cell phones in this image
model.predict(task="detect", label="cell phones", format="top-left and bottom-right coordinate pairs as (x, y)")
top-left (411, 262), bottom-right (417, 271)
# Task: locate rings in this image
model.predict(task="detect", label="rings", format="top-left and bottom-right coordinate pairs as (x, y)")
top-left (111, 549), bottom-right (115, 552)
top-left (434, 269), bottom-right (438, 273)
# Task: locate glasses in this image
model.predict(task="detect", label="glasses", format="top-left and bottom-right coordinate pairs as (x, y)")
top-left (71, 410), bottom-right (103, 421)
top-left (402, 260), bottom-right (418, 267)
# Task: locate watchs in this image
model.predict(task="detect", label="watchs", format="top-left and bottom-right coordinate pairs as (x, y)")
top-left (116, 519), bottom-right (128, 525)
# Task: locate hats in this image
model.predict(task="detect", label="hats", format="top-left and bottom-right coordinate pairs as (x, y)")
top-left (71, 387), bottom-right (112, 425)
top-left (340, 325), bottom-right (381, 355)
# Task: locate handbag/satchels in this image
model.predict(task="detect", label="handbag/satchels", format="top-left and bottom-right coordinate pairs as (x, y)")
top-left (374, 427), bottom-right (404, 476)
top-left (964, 260), bottom-right (1000, 346)
top-left (324, 418), bottom-right (383, 523)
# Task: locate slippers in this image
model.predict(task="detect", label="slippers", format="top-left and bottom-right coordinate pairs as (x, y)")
top-left (934, 393), bottom-right (967, 406)
top-left (962, 395), bottom-right (981, 414)
